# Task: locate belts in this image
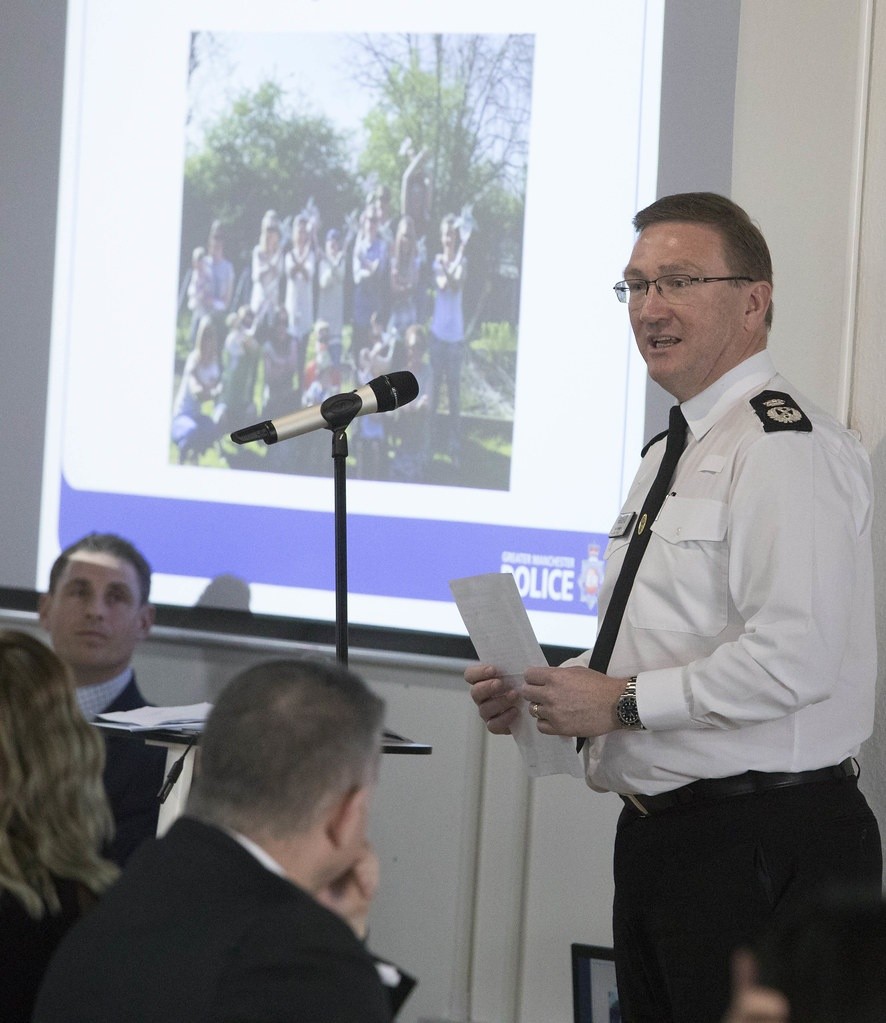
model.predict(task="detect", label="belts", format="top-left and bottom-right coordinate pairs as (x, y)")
top-left (619, 757), bottom-right (855, 819)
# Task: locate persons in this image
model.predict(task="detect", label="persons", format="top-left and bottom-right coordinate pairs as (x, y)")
top-left (0, 631), bottom-right (125, 1023)
top-left (464, 194), bottom-right (886, 1023)
top-left (27, 528), bottom-right (168, 860)
top-left (28, 659), bottom-right (417, 1023)
top-left (173, 183), bottom-right (482, 481)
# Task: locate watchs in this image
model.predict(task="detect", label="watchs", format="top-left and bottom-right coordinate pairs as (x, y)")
top-left (615, 676), bottom-right (645, 730)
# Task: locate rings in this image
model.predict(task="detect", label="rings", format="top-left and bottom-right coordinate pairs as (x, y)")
top-left (534, 705), bottom-right (541, 719)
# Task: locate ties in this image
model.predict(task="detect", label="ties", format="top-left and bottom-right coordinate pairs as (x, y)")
top-left (576, 405), bottom-right (688, 754)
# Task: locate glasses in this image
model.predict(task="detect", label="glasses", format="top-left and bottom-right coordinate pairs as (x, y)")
top-left (613, 274), bottom-right (753, 303)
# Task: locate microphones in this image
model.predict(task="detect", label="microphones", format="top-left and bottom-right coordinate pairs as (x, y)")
top-left (230, 370), bottom-right (420, 446)
